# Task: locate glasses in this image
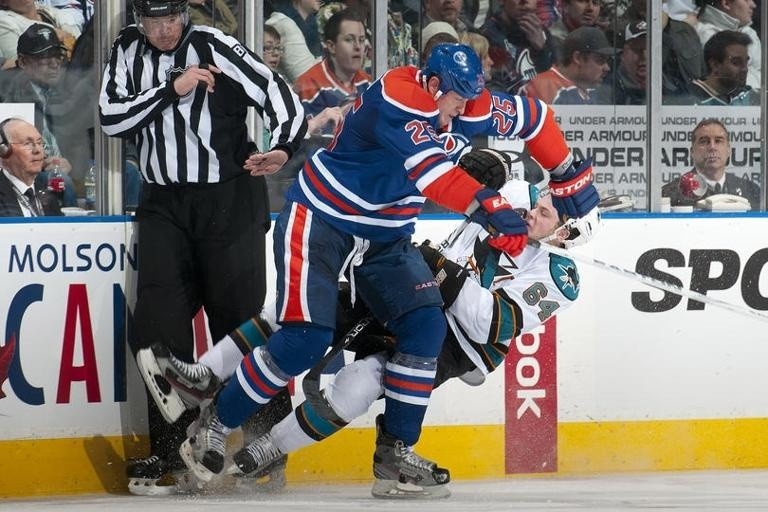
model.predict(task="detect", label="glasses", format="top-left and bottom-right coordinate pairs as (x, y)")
top-left (263, 44), bottom-right (286, 55)
top-left (22, 53), bottom-right (64, 66)
top-left (4, 139), bottom-right (49, 152)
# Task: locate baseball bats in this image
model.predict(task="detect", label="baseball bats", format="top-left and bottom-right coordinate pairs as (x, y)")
top-left (301, 222), bottom-right (469, 419)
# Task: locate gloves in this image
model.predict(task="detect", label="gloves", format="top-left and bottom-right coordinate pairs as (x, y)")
top-left (473, 187), bottom-right (530, 257)
top-left (546, 161), bottom-right (600, 219)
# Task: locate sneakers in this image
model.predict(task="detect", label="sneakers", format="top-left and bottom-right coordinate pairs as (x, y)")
top-left (125, 446), bottom-right (187, 480)
top-left (233, 429), bottom-right (288, 477)
top-left (185, 405), bottom-right (231, 473)
top-left (153, 343), bottom-right (224, 409)
top-left (373, 439), bottom-right (451, 486)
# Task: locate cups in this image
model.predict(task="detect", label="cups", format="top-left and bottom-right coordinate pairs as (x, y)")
top-left (661, 197), bottom-right (671, 213)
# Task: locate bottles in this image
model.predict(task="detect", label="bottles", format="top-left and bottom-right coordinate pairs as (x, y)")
top-left (47, 160), bottom-right (64, 216)
top-left (85, 161), bottom-right (98, 210)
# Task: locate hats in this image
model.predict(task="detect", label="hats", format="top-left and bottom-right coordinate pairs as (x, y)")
top-left (562, 25), bottom-right (624, 57)
top-left (419, 20), bottom-right (460, 49)
top-left (621, 18), bottom-right (648, 38)
top-left (18, 23), bottom-right (68, 56)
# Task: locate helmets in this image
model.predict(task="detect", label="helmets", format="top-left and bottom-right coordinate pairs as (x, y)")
top-left (132, 0), bottom-right (189, 21)
top-left (549, 189), bottom-right (601, 251)
top-left (425, 43), bottom-right (486, 99)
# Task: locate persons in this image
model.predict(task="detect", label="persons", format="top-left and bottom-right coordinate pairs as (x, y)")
top-left (98, 0), bottom-right (308, 498)
top-left (598, 0), bottom-right (762, 105)
top-left (313, 0), bottom-right (419, 75)
top-left (188, 0), bottom-right (374, 211)
top-left (0, 0), bottom-right (102, 196)
top-left (391, 0), bottom-right (647, 105)
top-left (662, 120), bottom-right (760, 210)
top-left (136, 186), bottom-right (599, 479)
top-left (179, 43), bottom-right (602, 502)
top-left (0, 118), bottom-right (65, 218)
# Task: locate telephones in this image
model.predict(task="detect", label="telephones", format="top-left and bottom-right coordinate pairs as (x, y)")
top-left (696, 193), bottom-right (752, 213)
top-left (598, 195), bottom-right (635, 213)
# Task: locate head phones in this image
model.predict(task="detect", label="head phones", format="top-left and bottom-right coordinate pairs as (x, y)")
top-left (0, 120), bottom-right (12, 159)
